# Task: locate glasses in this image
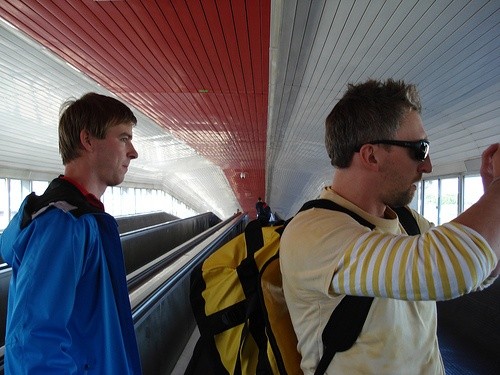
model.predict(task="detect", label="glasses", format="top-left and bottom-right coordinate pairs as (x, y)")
top-left (356, 139), bottom-right (430, 162)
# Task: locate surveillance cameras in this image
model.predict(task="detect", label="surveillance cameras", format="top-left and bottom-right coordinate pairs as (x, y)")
top-left (240, 174), bottom-right (245, 179)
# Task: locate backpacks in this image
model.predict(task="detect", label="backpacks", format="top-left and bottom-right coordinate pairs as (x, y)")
top-left (190, 198), bottom-right (421, 375)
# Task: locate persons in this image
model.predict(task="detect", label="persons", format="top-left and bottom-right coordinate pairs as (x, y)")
top-left (279, 80), bottom-right (500, 375)
top-left (256, 197), bottom-right (264, 216)
top-left (0, 94), bottom-right (138, 375)
